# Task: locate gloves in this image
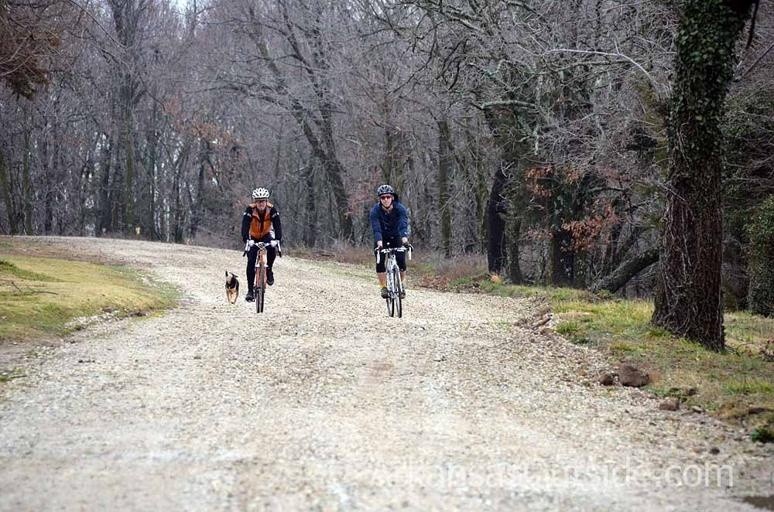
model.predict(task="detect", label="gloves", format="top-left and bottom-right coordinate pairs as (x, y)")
top-left (374, 246), bottom-right (382, 254)
top-left (403, 242), bottom-right (414, 252)
top-left (249, 240), bottom-right (254, 247)
top-left (271, 240), bottom-right (277, 247)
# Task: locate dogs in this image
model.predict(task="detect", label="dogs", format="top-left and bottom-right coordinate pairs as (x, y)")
top-left (225, 270), bottom-right (240, 304)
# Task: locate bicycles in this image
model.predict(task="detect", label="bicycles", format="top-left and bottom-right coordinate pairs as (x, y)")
top-left (376, 246), bottom-right (412, 318)
top-left (243, 240), bottom-right (282, 313)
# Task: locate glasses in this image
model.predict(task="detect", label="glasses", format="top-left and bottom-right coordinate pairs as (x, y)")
top-left (381, 196), bottom-right (392, 200)
top-left (256, 200), bottom-right (266, 203)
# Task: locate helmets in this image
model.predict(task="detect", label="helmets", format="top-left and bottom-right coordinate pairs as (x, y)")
top-left (252, 187), bottom-right (269, 199)
top-left (377, 185), bottom-right (394, 196)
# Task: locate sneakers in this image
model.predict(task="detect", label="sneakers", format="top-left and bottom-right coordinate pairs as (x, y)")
top-left (266, 267), bottom-right (274, 285)
top-left (400, 287), bottom-right (406, 298)
top-left (380, 287), bottom-right (388, 298)
top-left (246, 292), bottom-right (254, 300)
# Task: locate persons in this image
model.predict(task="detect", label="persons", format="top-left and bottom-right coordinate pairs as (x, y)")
top-left (241, 187), bottom-right (281, 302)
top-left (370, 185), bottom-right (409, 298)
